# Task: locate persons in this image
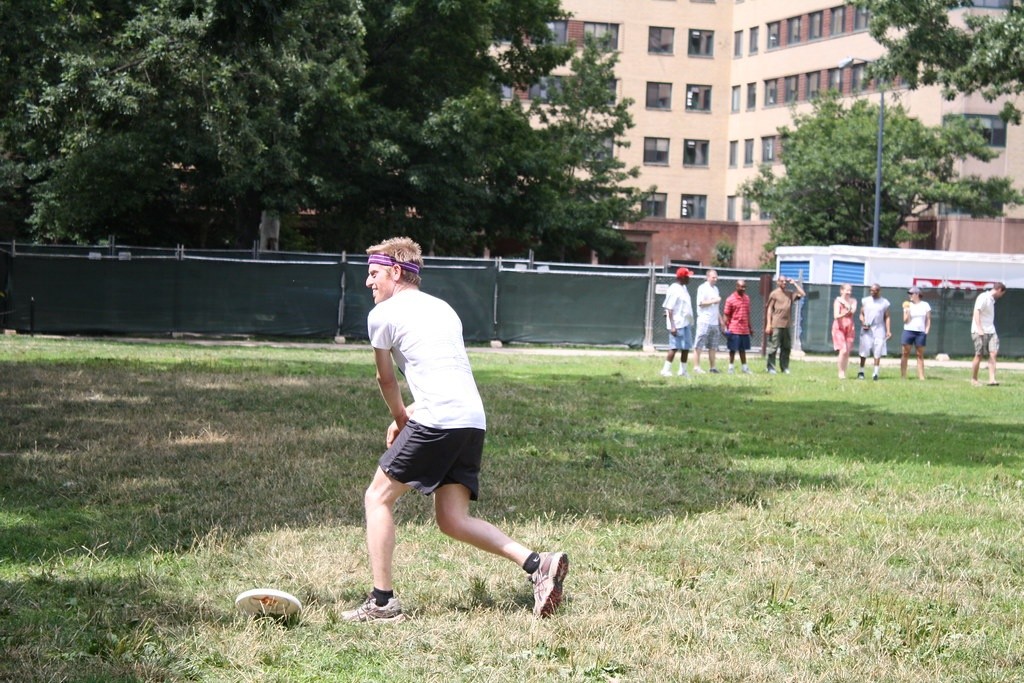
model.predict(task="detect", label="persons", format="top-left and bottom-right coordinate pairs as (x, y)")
top-left (724, 279), bottom-right (754, 375)
top-left (694, 269), bottom-right (727, 375)
top-left (334, 237), bottom-right (569, 619)
top-left (764, 275), bottom-right (806, 376)
top-left (659, 267), bottom-right (694, 378)
top-left (831, 283), bottom-right (858, 381)
top-left (969, 281), bottom-right (1005, 388)
top-left (858, 284), bottom-right (892, 380)
top-left (900, 287), bottom-right (931, 382)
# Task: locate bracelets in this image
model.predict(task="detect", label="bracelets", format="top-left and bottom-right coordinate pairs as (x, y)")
top-left (395, 408), bottom-right (407, 421)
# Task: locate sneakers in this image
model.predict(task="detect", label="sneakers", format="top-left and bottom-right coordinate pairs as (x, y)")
top-left (527, 551), bottom-right (569, 618)
top-left (339, 595), bottom-right (406, 626)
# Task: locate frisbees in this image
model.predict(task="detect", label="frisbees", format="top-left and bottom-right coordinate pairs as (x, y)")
top-left (235, 588), bottom-right (302, 617)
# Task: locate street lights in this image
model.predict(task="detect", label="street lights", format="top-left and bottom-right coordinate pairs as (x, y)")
top-left (838, 57), bottom-right (886, 247)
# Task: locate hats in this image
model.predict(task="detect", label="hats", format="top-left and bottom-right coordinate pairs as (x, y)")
top-left (676, 267), bottom-right (694, 279)
top-left (907, 287), bottom-right (923, 297)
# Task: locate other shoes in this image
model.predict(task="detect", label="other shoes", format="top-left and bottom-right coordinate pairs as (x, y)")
top-left (986, 379), bottom-right (999, 387)
top-left (872, 373), bottom-right (878, 381)
top-left (858, 372), bottom-right (865, 380)
top-left (660, 364), bottom-right (791, 377)
